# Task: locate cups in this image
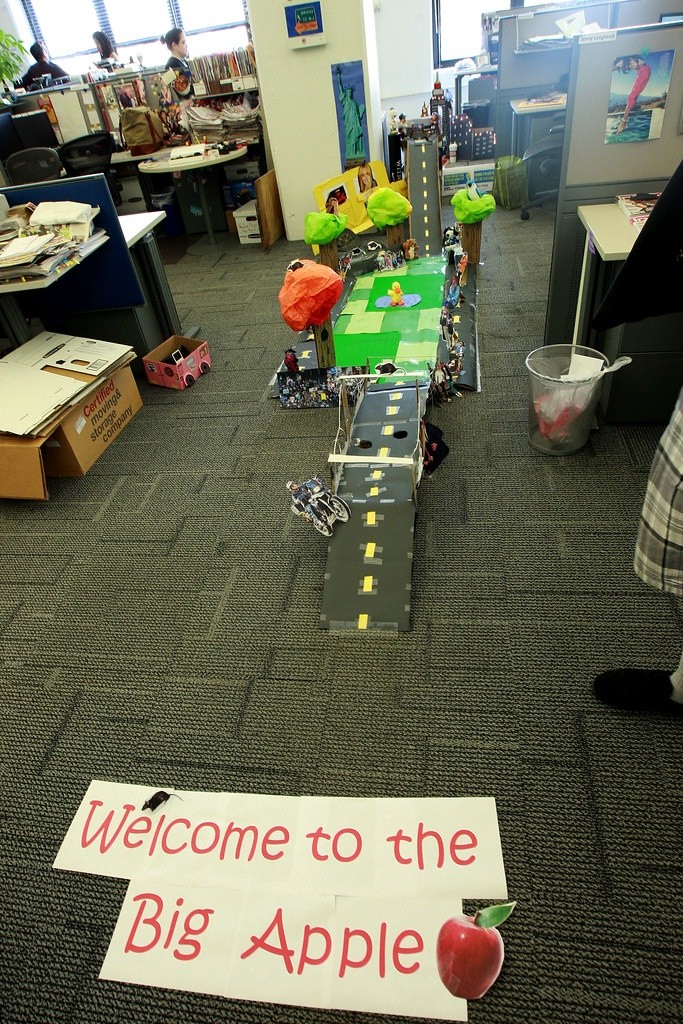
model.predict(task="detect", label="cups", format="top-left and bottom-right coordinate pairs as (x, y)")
top-left (449, 144), bottom-right (458, 163)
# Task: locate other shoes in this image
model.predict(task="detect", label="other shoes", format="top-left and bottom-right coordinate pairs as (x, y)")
top-left (592, 669), bottom-right (683, 716)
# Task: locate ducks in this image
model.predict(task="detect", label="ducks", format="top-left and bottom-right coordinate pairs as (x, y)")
top-left (387, 282), bottom-right (403, 306)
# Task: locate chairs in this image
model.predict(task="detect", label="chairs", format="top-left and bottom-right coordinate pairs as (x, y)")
top-left (4, 148), bottom-right (62, 187)
top-left (518, 112), bottom-right (566, 221)
top-left (59, 131), bottom-right (113, 199)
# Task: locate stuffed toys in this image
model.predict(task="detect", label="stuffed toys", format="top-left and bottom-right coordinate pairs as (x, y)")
top-left (442, 227), bottom-right (457, 265)
top-left (387, 281), bottom-right (405, 307)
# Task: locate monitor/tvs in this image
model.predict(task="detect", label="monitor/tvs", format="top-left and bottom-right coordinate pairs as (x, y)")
top-left (11, 109), bottom-right (61, 150)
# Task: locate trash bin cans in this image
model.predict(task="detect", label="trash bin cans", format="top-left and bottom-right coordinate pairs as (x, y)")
top-left (526, 344), bottom-right (610, 457)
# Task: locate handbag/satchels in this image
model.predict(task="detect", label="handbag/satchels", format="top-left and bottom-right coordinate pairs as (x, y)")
top-left (120, 106), bottom-right (163, 156)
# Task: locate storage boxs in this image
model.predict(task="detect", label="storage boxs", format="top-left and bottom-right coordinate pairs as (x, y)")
top-left (232, 200), bottom-right (262, 245)
top-left (44, 368), bottom-right (143, 474)
top-left (142, 334), bottom-right (213, 390)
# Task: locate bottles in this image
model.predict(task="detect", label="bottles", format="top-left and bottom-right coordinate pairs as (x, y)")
top-left (432, 81), bottom-right (444, 95)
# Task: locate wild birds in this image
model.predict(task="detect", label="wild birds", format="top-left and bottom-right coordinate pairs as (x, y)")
top-left (468, 180), bottom-right (480, 201)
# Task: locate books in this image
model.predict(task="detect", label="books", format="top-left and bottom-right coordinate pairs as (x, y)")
top-left (0, 193), bottom-right (99, 280)
top-left (166, 98), bottom-right (264, 164)
top-left (140, 46), bottom-right (256, 98)
top-left (16, 66), bottom-right (111, 90)
top-left (614, 192), bottom-right (663, 237)
top-left (518, 96), bottom-right (565, 110)
top-left (518, 9), bottom-right (600, 48)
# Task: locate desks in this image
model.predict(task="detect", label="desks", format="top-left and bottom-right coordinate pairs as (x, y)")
top-left (572, 199), bottom-right (683, 396)
top-left (0, 231), bottom-right (110, 345)
top-left (454, 63), bottom-right (498, 118)
top-left (52, 124), bottom-right (261, 213)
top-left (509, 92), bottom-right (568, 200)
top-left (137, 141), bottom-right (248, 254)
top-left (118, 210), bottom-right (201, 359)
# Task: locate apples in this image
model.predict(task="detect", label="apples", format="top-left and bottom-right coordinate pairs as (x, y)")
top-left (436, 910), bottom-right (505, 999)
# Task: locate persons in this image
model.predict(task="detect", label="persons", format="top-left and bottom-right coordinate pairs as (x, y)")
top-left (285, 480), bottom-right (312, 511)
top-left (22, 41), bottom-right (69, 90)
top-left (398, 113), bottom-right (408, 130)
top-left (160, 29), bottom-right (196, 100)
top-left (339, 253), bottom-right (350, 283)
top-left (358, 161), bottom-right (378, 193)
top-left (610, 55), bottom-right (651, 134)
top-left (434, 250), bottom-right (468, 403)
top-left (91, 31), bottom-right (118, 63)
top-left (119, 86), bottom-right (132, 108)
top-left (335, 190), bottom-right (346, 205)
top-left (592, 145), bottom-right (683, 719)
top-left (285, 349), bottom-right (299, 371)
